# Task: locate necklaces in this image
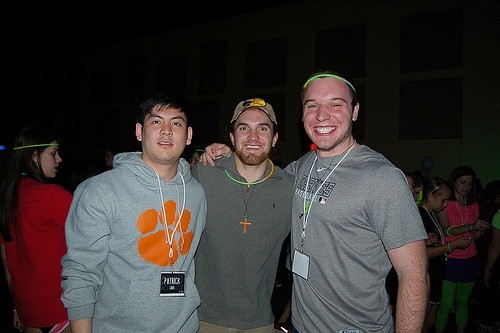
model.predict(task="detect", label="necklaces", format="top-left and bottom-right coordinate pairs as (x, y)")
top-left (238, 175), bottom-right (263, 233)
top-left (305, 141), bottom-right (354, 214)
top-left (224, 153), bottom-right (275, 185)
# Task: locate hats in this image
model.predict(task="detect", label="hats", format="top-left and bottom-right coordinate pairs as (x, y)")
top-left (231, 99), bottom-right (277, 125)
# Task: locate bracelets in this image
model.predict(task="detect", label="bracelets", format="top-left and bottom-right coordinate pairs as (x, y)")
top-left (445, 241), bottom-right (452, 254)
top-left (466, 223), bottom-right (472, 232)
top-left (447, 227), bottom-right (453, 237)
top-left (274, 320), bottom-right (291, 330)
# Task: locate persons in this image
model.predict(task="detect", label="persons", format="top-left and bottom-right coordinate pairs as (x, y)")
top-left (470, 177), bottom-right (500, 333)
top-left (0, 69), bottom-right (405, 333)
top-left (191, 97), bottom-right (295, 332)
top-left (415, 178), bottom-right (472, 333)
top-left (432, 166), bottom-right (490, 333)
top-left (198, 71), bottom-right (431, 333)
top-left (403, 169), bottom-right (436, 207)
top-left (61, 91), bottom-right (207, 333)
